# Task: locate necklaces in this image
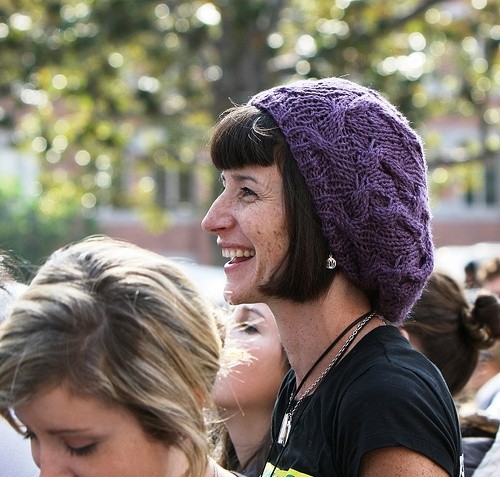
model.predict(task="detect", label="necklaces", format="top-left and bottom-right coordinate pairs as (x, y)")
top-left (260, 312), bottom-right (374, 477)
top-left (276, 311), bottom-right (376, 447)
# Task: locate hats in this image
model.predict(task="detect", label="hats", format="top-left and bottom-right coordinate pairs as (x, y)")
top-left (250, 78), bottom-right (434, 321)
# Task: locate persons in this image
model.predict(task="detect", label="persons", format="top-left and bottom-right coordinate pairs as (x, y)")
top-left (202, 78), bottom-right (461, 477)
top-left (0, 235), bottom-right (500, 477)
